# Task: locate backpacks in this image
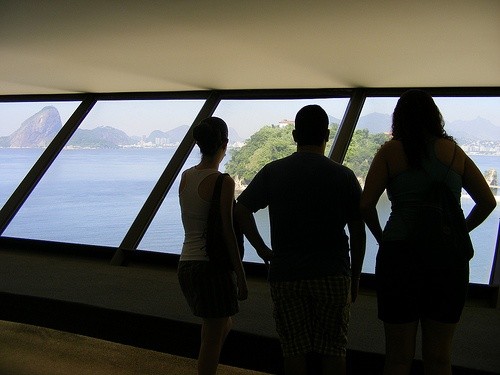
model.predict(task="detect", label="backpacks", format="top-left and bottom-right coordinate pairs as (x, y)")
top-left (387, 142), bottom-right (472, 270)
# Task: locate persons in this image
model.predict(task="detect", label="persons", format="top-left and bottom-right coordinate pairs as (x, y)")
top-left (360, 88), bottom-right (497, 375)
top-left (234, 105), bottom-right (366, 375)
top-left (177, 117), bottom-right (248, 375)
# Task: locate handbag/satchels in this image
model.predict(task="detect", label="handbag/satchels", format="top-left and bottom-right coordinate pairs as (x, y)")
top-left (207, 174), bottom-right (244, 266)
